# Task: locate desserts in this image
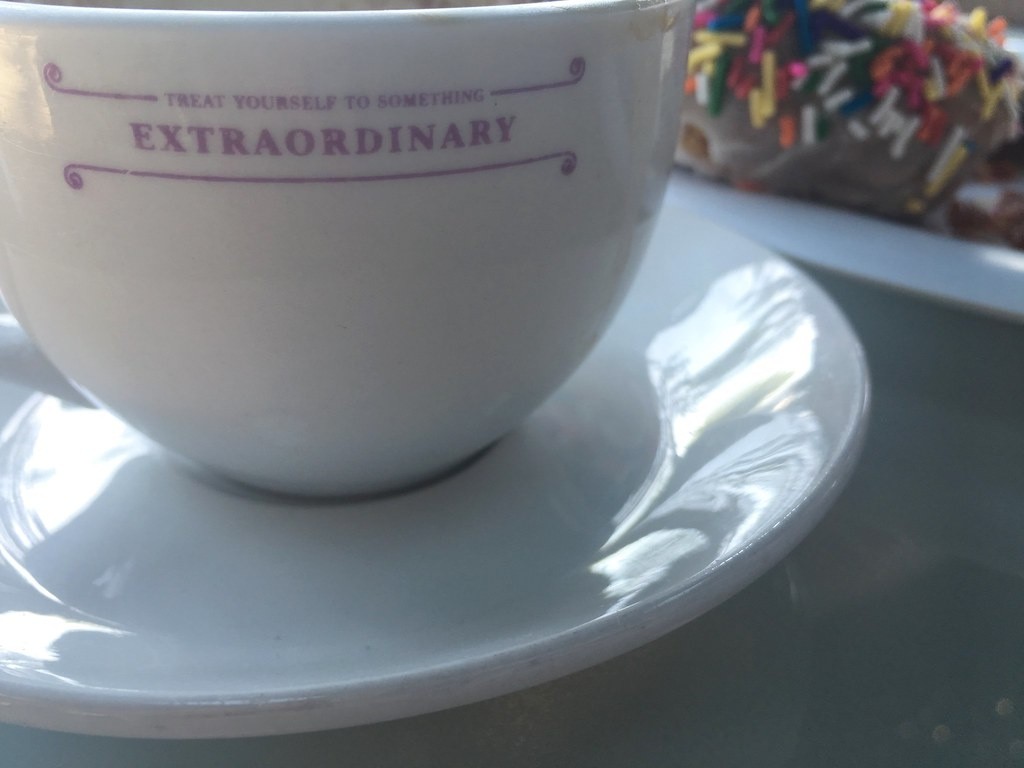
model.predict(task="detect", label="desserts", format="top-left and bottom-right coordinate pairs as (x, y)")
top-left (661, 0), bottom-right (1024, 254)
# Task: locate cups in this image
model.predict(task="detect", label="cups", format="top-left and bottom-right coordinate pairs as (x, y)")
top-left (0, 1), bottom-right (694, 495)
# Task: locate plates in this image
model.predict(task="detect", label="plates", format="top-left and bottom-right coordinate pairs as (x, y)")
top-left (0, 199), bottom-right (875, 733)
